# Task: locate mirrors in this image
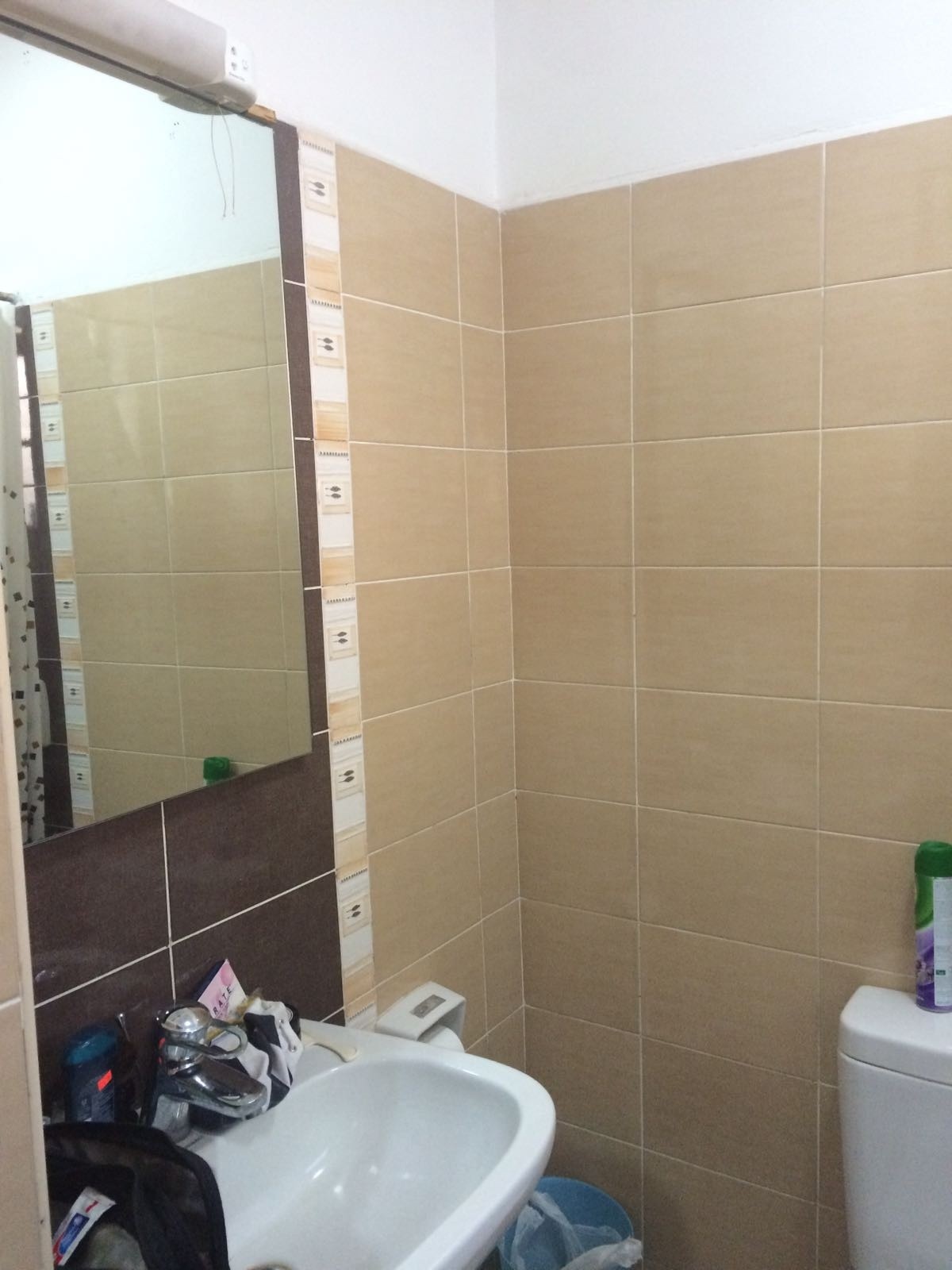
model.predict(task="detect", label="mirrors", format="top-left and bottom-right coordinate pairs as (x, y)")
top-left (1, 17), bottom-right (315, 848)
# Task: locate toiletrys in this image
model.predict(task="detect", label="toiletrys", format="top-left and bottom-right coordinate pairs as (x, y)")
top-left (53, 1185), bottom-right (119, 1269)
top-left (54, 1021), bottom-right (127, 1137)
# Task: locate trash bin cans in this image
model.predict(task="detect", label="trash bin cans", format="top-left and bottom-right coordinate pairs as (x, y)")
top-left (492, 1173), bottom-right (640, 1270)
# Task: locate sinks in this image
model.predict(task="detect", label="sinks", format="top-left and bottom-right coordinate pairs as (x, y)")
top-left (106, 1006), bottom-right (559, 1269)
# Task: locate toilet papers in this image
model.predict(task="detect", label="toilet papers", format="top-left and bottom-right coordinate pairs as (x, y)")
top-left (418, 1023), bottom-right (468, 1057)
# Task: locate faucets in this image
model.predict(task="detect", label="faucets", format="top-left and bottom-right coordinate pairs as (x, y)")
top-left (148, 1002), bottom-right (272, 1153)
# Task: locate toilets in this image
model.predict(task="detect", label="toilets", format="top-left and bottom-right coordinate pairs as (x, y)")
top-left (832, 978), bottom-right (951, 1270)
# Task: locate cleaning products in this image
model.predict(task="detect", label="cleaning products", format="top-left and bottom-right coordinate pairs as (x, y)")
top-left (906, 836), bottom-right (952, 1015)
top-left (202, 758), bottom-right (236, 788)
